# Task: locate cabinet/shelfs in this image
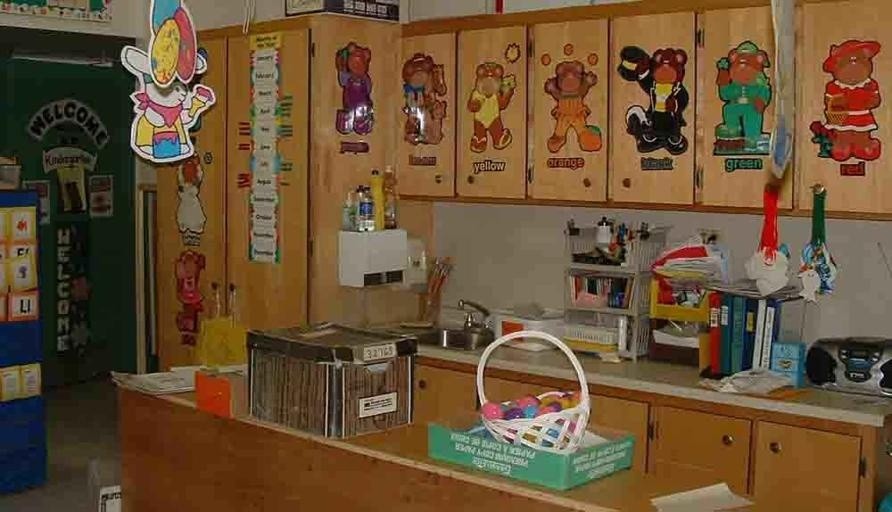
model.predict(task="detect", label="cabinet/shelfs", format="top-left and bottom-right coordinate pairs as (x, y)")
top-left (154, 20), bottom-right (402, 369)
top-left (530, 8), bottom-right (696, 210)
top-left (483, 364), bottom-right (650, 474)
top-left (415, 355), bottom-right (478, 424)
top-left (401, 25), bottom-right (529, 205)
top-left (701, 1), bottom-right (891, 220)
top-left (651, 394), bottom-right (865, 506)
top-left (0, 186), bottom-right (49, 494)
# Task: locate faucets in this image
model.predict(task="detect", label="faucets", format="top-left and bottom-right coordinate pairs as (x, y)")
top-left (456, 299), bottom-right (488, 317)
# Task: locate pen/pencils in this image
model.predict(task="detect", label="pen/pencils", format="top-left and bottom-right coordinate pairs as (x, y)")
top-left (614, 222), bottom-right (635, 245)
top-left (427, 255), bottom-right (453, 292)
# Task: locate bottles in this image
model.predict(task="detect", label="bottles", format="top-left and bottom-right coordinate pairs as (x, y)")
top-left (342, 162), bottom-right (400, 233)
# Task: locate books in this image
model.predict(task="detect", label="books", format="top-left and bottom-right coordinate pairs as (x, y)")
top-left (708, 291), bottom-right (777, 378)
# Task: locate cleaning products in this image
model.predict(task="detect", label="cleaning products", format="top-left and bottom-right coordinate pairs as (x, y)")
top-left (384, 163), bottom-right (398, 229)
top-left (370, 169), bottom-right (384, 231)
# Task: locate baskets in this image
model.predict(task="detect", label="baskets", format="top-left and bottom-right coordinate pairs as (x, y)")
top-left (477, 330), bottom-right (593, 455)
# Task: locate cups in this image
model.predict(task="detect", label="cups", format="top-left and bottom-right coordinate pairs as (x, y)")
top-left (414, 285), bottom-right (443, 322)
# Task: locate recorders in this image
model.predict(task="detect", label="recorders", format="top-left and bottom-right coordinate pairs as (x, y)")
top-left (806, 336), bottom-right (892, 400)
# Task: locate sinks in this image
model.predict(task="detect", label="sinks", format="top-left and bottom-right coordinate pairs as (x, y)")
top-left (402, 326), bottom-right (467, 351)
